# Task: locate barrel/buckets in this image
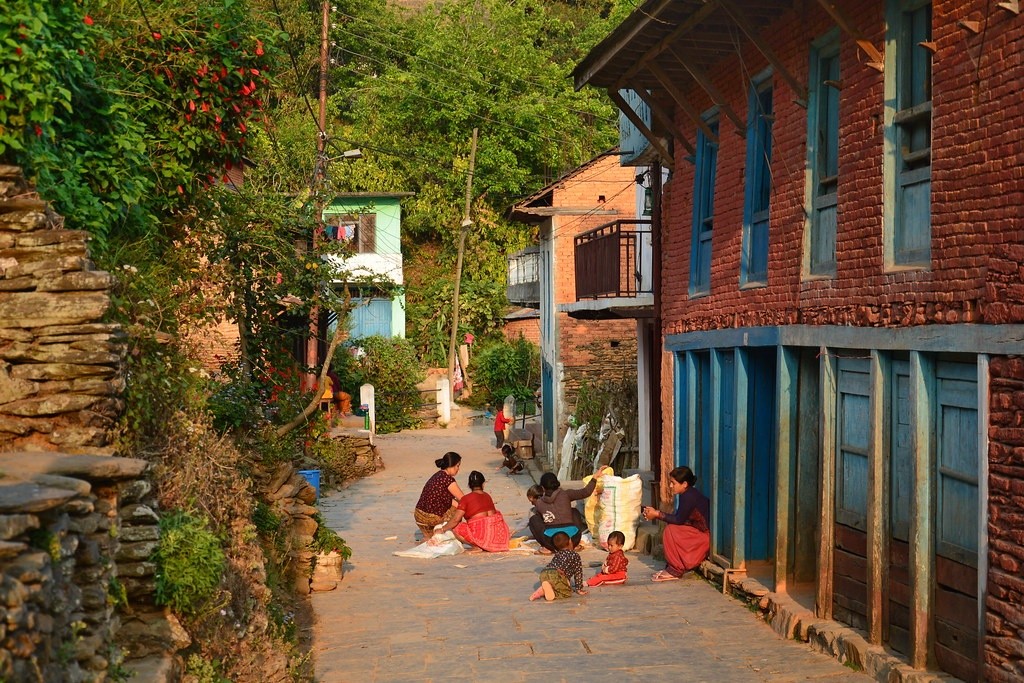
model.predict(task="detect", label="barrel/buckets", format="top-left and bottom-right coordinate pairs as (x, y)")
top-left (299, 470), bottom-right (321, 499)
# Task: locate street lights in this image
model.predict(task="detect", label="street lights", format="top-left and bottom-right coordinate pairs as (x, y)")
top-left (307, 149), bottom-right (363, 388)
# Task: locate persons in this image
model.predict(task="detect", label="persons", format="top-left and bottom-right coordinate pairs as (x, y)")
top-left (317, 364), bottom-right (350, 419)
top-left (581, 531), bottom-right (629, 587)
top-left (643, 465), bottom-right (710, 582)
top-left (530, 465), bottom-right (608, 555)
top-left (414, 451), bottom-right (464, 538)
top-left (494, 403), bottom-right (511, 450)
top-left (530, 531), bottom-right (590, 601)
top-left (535, 386), bottom-right (542, 412)
top-left (495, 444), bottom-right (524, 475)
top-left (510, 484), bottom-right (544, 538)
top-left (434, 470), bottom-right (510, 554)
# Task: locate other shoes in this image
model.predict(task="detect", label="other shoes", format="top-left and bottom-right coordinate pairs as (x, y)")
top-left (530, 586), bottom-right (545, 601)
top-left (509, 472), bottom-right (515, 475)
top-left (542, 581), bottom-right (555, 601)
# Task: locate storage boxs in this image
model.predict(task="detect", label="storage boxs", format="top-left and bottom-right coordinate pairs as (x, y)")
top-left (514, 432), bottom-right (535, 459)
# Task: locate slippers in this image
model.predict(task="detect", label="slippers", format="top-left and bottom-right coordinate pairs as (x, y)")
top-left (534, 549), bottom-right (552, 555)
top-left (651, 570), bottom-right (679, 581)
top-left (573, 545), bottom-right (584, 551)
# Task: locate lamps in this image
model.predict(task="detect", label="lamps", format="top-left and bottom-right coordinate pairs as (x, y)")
top-left (642, 185), bottom-right (653, 216)
